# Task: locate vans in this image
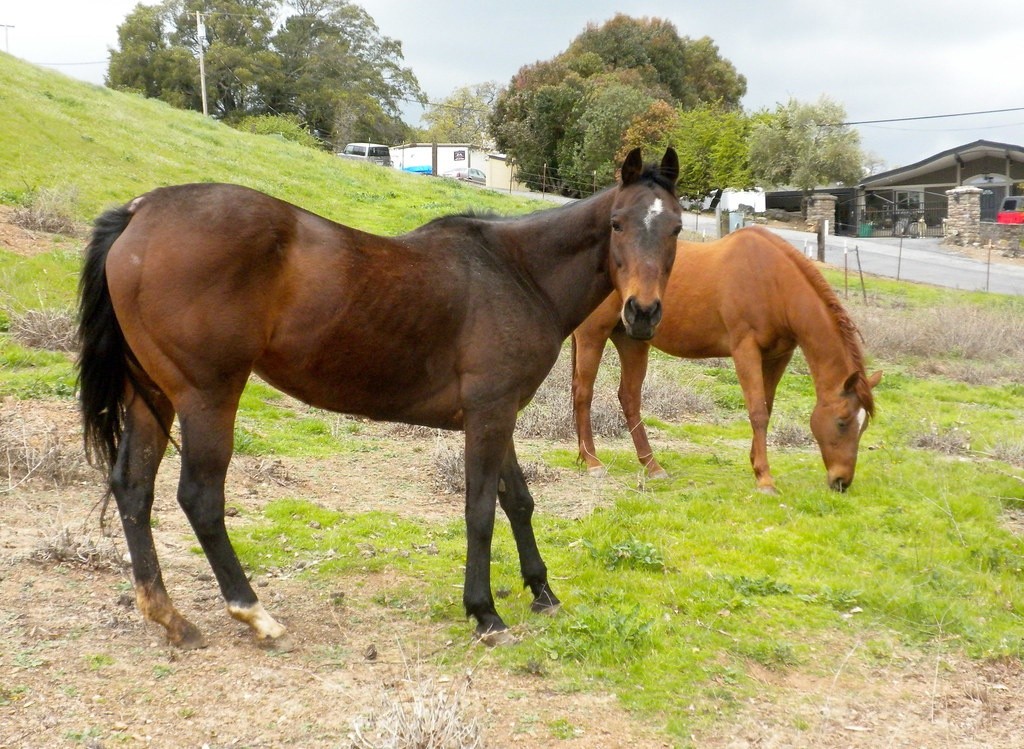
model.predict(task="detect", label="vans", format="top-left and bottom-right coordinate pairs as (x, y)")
top-left (336, 143), bottom-right (391, 168)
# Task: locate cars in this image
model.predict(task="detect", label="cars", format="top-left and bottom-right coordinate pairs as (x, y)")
top-left (442, 167), bottom-right (487, 186)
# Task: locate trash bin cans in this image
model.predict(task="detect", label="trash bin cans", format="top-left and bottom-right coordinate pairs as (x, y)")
top-left (859, 223), bottom-right (873, 237)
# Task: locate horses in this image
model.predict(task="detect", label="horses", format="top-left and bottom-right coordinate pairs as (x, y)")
top-left (70, 144), bottom-right (685, 651)
top-left (568, 225), bottom-right (884, 494)
